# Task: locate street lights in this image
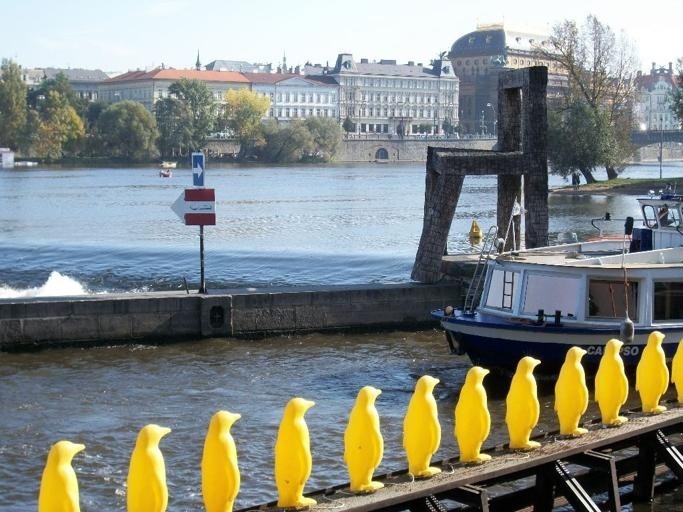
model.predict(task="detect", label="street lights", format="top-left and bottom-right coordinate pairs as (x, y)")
top-left (486, 102), bottom-right (497, 135)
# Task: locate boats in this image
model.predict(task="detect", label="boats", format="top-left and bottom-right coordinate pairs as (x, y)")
top-left (429, 181), bottom-right (683, 376)
top-left (159, 170), bottom-right (172, 178)
top-left (159, 160), bottom-right (178, 168)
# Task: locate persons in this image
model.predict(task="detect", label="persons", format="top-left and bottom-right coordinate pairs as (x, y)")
top-left (658, 204), bottom-right (668, 226)
